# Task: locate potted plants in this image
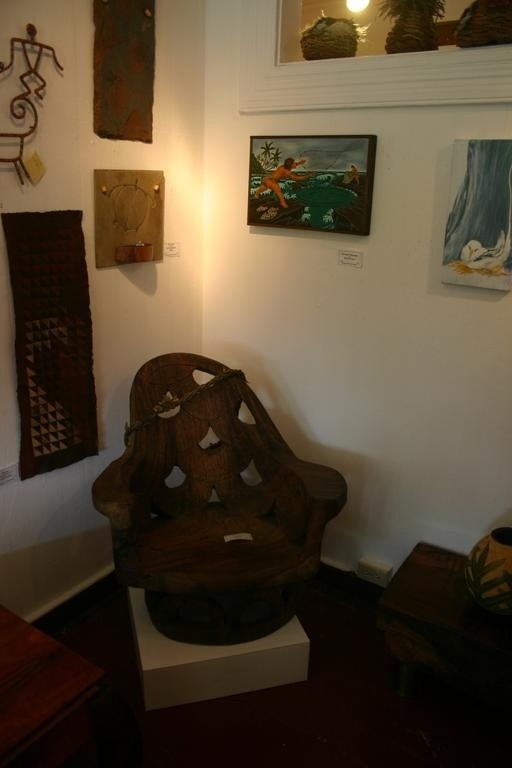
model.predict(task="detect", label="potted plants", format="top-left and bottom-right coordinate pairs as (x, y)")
top-left (377, 0), bottom-right (448, 54)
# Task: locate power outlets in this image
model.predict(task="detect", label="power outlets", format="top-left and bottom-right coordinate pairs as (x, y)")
top-left (165, 240), bottom-right (181, 257)
top-left (356, 558), bottom-right (393, 588)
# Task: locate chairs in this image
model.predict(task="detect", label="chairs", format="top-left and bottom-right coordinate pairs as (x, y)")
top-left (91, 353), bottom-right (348, 646)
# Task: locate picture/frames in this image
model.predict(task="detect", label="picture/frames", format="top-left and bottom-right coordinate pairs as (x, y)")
top-left (247, 134), bottom-right (377, 236)
